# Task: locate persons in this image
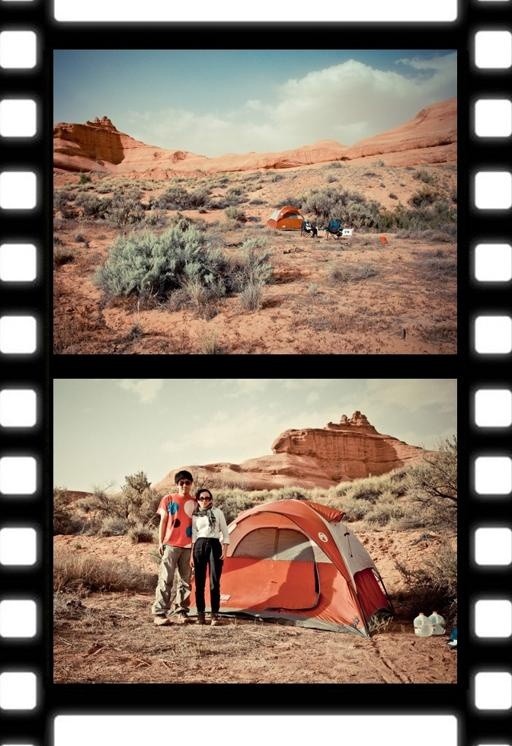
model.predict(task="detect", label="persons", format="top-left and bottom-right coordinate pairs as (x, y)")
top-left (305, 219), bottom-right (318, 237)
top-left (150, 470), bottom-right (199, 626)
top-left (188, 489), bottom-right (231, 626)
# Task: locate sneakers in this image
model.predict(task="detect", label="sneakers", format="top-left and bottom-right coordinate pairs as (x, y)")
top-left (196, 612), bottom-right (205, 624)
top-left (153, 614), bottom-right (174, 626)
top-left (211, 612), bottom-right (219, 626)
top-left (178, 612), bottom-right (191, 624)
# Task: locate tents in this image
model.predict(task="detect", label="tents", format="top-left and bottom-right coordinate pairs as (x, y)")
top-left (267, 203), bottom-right (306, 231)
top-left (178, 497), bottom-right (396, 638)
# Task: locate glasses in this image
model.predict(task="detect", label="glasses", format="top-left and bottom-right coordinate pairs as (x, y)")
top-left (197, 496), bottom-right (212, 502)
top-left (178, 480), bottom-right (192, 487)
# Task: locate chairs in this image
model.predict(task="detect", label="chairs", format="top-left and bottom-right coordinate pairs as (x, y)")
top-left (300, 218), bottom-right (344, 240)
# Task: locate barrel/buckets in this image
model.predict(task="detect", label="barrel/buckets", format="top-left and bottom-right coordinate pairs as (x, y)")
top-left (429, 610), bottom-right (446, 634)
top-left (413, 612), bottom-right (434, 637)
